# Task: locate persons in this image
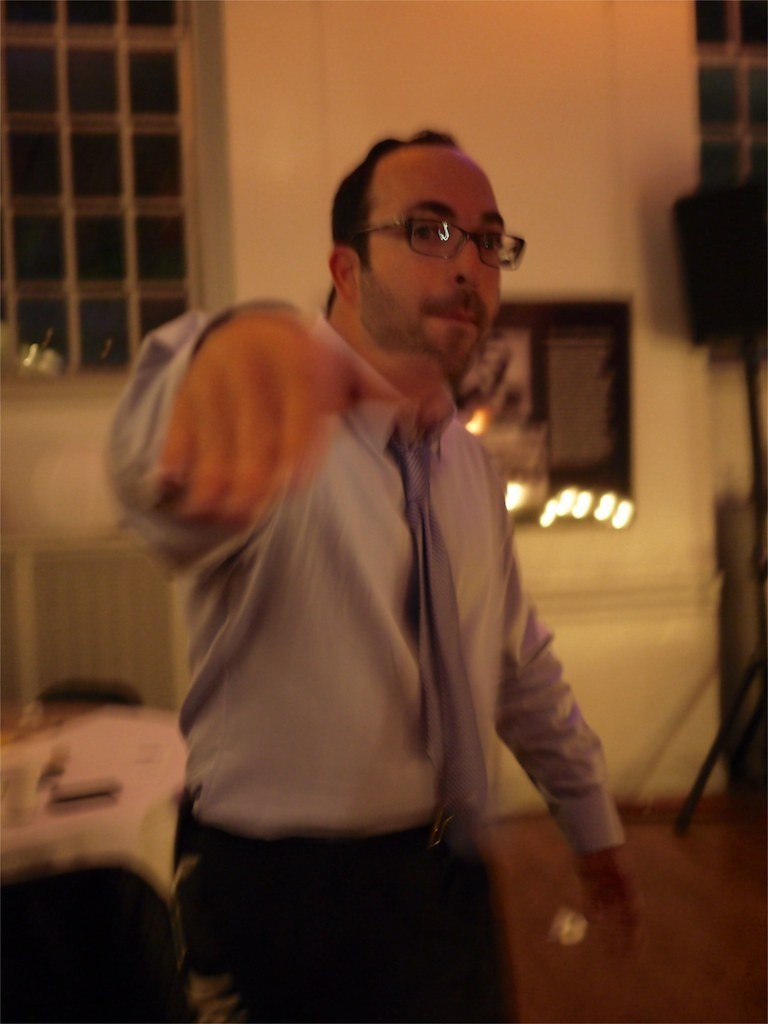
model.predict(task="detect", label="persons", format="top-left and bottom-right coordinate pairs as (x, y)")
top-left (103, 129), bottom-right (641, 1024)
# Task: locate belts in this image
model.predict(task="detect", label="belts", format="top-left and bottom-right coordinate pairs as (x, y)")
top-left (191, 809), bottom-right (461, 864)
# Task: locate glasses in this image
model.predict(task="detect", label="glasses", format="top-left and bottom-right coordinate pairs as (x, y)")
top-left (347, 217), bottom-right (528, 271)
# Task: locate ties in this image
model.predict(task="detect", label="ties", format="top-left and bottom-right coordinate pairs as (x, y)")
top-left (387, 430), bottom-right (489, 853)
